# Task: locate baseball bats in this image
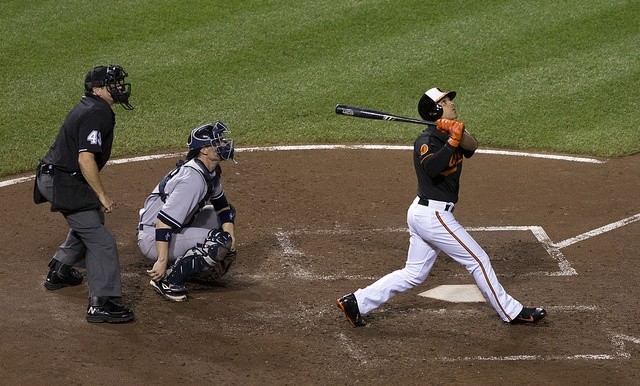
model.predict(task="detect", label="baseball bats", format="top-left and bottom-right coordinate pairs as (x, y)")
top-left (335, 105), bottom-right (465, 134)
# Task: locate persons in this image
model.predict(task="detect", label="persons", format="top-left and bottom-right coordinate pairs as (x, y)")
top-left (32, 64), bottom-right (137, 325)
top-left (136, 120), bottom-right (239, 304)
top-left (335, 86), bottom-right (549, 328)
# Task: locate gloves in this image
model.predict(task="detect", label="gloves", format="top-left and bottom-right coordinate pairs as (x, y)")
top-left (447, 121), bottom-right (464, 147)
top-left (436, 119), bottom-right (456, 134)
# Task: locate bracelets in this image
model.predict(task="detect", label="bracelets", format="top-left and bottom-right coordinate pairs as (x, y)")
top-left (155, 228), bottom-right (173, 242)
top-left (217, 210), bottom-right (237, 227)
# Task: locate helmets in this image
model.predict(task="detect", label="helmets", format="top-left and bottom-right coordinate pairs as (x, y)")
top-left (84, 65), bottom-right (135, 110)
top-left (418, 87), bottom-right (457, 122)
top-left (187, 121), bottom-right (238, 166)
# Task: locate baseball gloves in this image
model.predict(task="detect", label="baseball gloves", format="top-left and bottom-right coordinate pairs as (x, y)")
top-left (204, 249), bottom-right (236, 283)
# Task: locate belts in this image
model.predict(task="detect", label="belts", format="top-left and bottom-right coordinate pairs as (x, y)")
top-left (419, 199), bottom-right (456, 213)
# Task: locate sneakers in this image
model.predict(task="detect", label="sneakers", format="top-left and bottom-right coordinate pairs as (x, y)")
top-left (86, 297), bottom-right (135, 324)
top-left (510, 307), bottom-right (546, 325)
top-left (149, 275), bottom-right (188, 303)
top-left (337, 293), bottom-right (366, 327)
top-left (44, 259), bottom-right (83, 290)
